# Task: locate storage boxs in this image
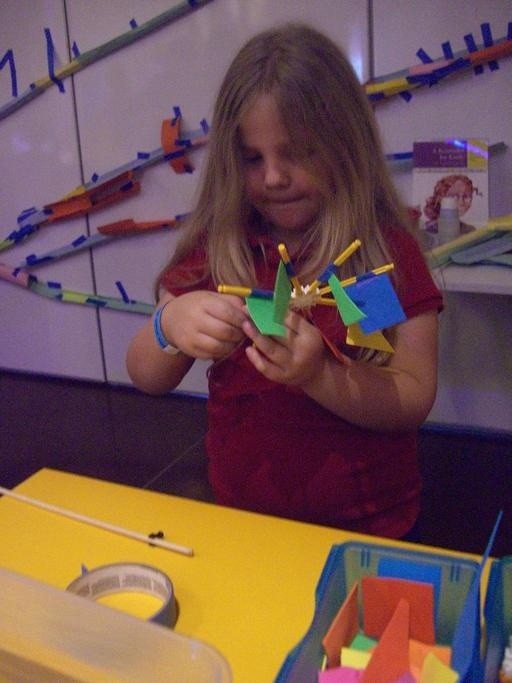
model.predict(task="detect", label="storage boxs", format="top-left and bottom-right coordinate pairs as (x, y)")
top-left (482, 555), bottom-right (511, 683)
top-left (273, 539), bottom-right (482, 683)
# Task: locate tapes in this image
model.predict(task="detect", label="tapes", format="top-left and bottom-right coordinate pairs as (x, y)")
top-left (65, 562), bottom-right (177, 633)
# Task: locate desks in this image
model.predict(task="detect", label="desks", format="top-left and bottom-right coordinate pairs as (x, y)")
top-left (432, 261), bottom-right (512, 296)
top-left (0, 467), bottom-right (511, 683)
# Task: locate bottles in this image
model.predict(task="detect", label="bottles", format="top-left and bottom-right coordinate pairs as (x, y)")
top-left (438, 197), bottom-right (460, 241)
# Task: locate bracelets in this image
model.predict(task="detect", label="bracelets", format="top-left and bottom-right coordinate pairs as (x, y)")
top-left (154, 300), bottom-right (180, 358)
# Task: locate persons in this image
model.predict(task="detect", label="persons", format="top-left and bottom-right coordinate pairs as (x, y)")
top-left (124, 19), bottom-right (446, 543)
